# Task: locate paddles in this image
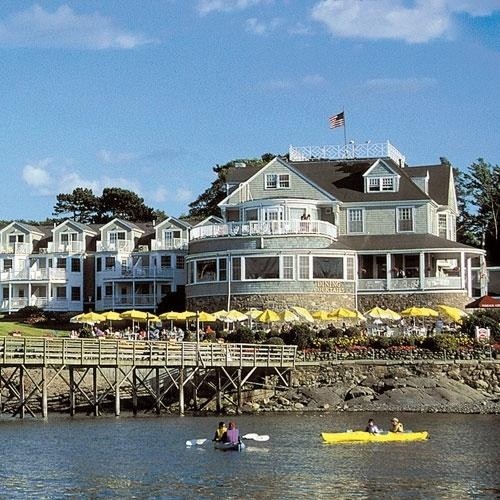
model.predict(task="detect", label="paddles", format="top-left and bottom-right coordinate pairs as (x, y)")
top-left (185, 433), bottom-right (270, 446)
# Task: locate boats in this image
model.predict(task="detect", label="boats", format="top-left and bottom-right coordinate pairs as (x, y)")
top-left (214, 442), bottom-right (245, 453)
top-left (321, 431), bottom-right (428, 443)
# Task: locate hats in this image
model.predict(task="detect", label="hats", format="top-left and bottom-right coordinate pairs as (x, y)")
top-left (368, 418), bottom-right (374, 423)
top-left (390, 417), bottom-right (399, 422)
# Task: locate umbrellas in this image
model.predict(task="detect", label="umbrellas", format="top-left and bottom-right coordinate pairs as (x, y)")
top-left (465, 295), bottom-right (500, 308)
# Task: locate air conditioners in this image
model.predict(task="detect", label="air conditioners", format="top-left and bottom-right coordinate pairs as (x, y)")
top-left (139, 245), bottom-right (149, 253)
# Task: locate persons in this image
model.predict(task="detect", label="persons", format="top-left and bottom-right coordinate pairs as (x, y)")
top-left (70, 325), bottom-right (216, 342)
top-left (365, 417), bottom-right (404, 433)
top-left (396, 267), bottom-right (405, 278)
top-left (212, 421), bottom-right (242, 443)
top-left (427, 323), bottom-right (436, 339)
top-left (301, 214), bottom-right (310, 220)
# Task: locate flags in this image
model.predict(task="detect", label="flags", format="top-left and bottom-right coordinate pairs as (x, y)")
top-left (327, 111), bottom-right (345, 129)
